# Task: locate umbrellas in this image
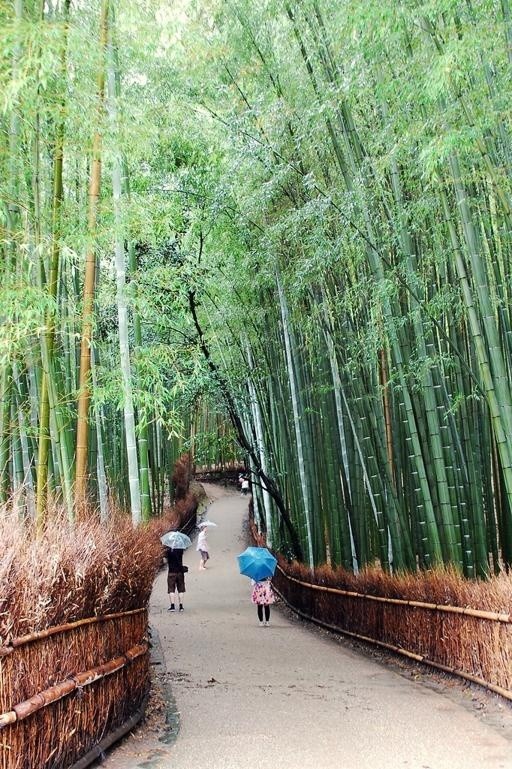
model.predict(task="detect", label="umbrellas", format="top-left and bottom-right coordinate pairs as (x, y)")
top-left (236, 546), bottom-right (279, 580)
top-left (196, 520), bottom-right (218, 529)
top-left (240, 479), bottom-right (249, 497)
top-left (159, 531), bottom-right (193, 550)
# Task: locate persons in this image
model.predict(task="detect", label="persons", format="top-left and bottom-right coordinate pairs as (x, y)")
top-left (162, 547), bottom-right (186, 612)
top-left (196, 526), bottom-right (211, 570)
top-left (250, 573), bottom-right (276, 626)
